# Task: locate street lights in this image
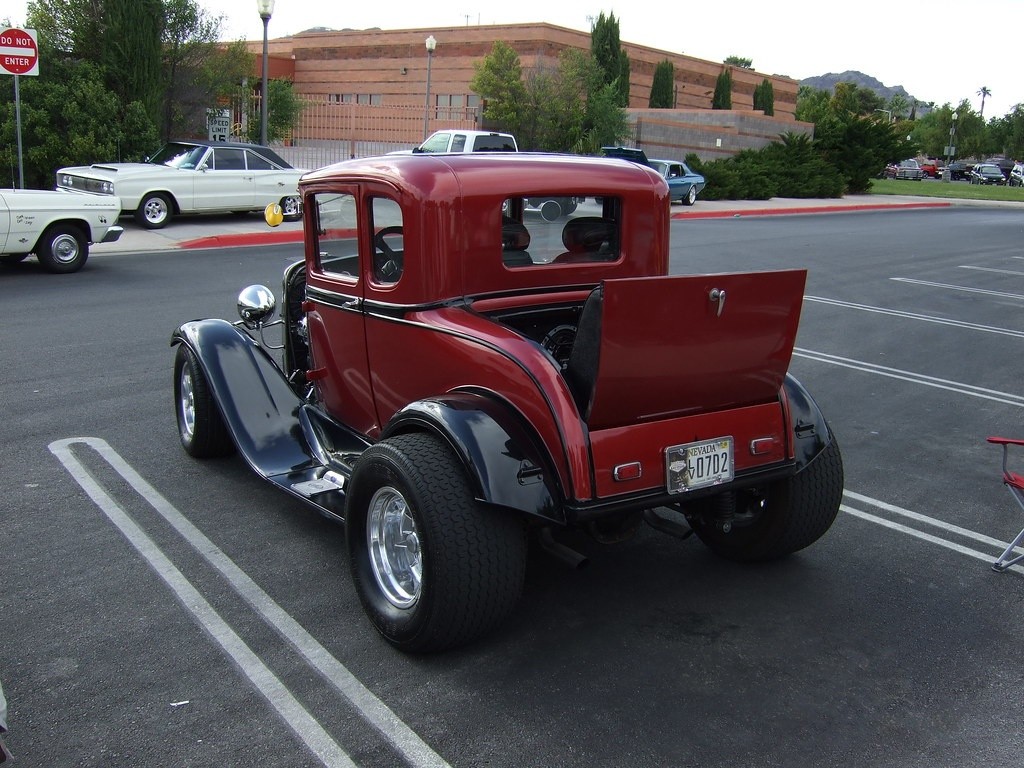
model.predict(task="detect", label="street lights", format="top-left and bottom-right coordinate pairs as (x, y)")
top-left (941, 111), bottom-right (959, 182)
top-left (253, 0), bottom-right (275, 150)
top-left (421, 35), bottom-right (438, 143)
top-left (874, 109), bottom-right (891, 127)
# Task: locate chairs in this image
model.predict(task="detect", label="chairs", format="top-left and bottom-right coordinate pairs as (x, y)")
top-left (551, 217), bottom-right (616, 264)
top-left (501, 214), bottom-right (532, 268)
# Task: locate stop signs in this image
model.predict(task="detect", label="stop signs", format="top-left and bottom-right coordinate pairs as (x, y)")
top-left (0, 30), bottom-right (40, 75)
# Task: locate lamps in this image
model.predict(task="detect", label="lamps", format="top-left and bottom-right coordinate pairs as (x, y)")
top-left (400, 67), bottom-right (407, 75)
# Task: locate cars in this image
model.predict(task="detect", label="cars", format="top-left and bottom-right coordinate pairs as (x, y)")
top-left (1008, 164), bottom-right (1024, 188)
top-left (911, 157), bottom-right (1017, 181)
top-left (595, 146), bottom-right (707, 206)
top-left (0, 183), bottom-right (124, 275)
top-left (882, 158), bottom-right (925, 182)
top-left (168, 148), bottom-right (844, 657)
top-left (56, 141), bottom-right (311, 230)
top-left (968, 164), bottom-right (1008, 186)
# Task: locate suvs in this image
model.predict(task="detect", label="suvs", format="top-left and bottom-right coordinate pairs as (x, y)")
top-left (386, 128), bottom-right (518, 160)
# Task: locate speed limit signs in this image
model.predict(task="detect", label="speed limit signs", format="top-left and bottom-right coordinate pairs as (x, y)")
top-left (208, 117), bottom-right (230, 144)
